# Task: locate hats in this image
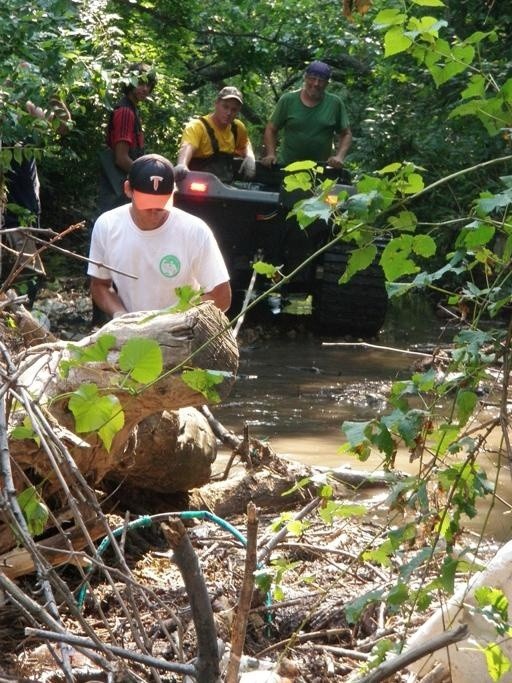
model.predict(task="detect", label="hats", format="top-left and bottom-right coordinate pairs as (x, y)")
top-left (218, 86), bottom-right (244, 106)
top-left (305, 60), bottom-right (331, 81)
top-left (127, 154), bottom-right (175, 212)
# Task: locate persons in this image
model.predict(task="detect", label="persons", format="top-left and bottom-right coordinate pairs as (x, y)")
top-left (260, 59), bottom-right (353, 169)
top-left (96, 64), bottom-right (159, 196)
top-left (84, 152), bottom-right (234, 321)
top-left (0, 60), bottom-right (73, 228)
top-left (174, 86), bottom-right (257, 183)
